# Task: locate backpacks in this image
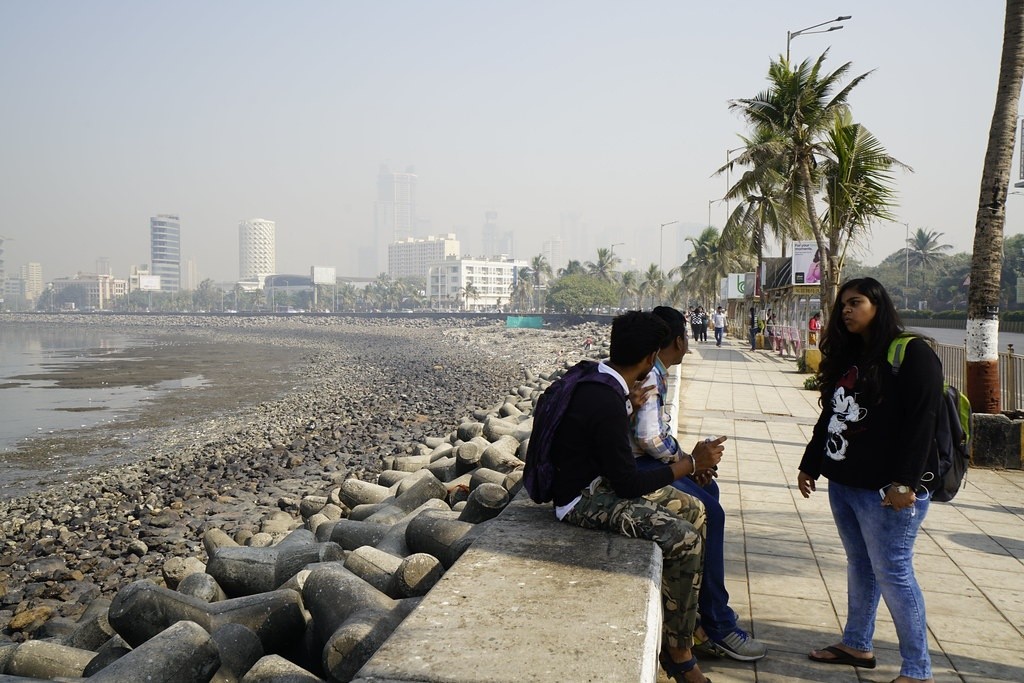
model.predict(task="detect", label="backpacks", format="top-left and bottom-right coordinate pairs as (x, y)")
top-left (887, 333), bottom-right (974, 502)
top-left (522, 361), bottom-right (625, 505)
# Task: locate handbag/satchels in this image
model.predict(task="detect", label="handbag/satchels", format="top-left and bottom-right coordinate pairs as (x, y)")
top-left (757, 316), bottom-right (764, 329)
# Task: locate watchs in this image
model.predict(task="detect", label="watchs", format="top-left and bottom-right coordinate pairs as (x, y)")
top-left (891, 484), bottom-right (912, 494)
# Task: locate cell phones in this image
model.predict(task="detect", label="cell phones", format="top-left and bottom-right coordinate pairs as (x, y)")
top-left (880, 483), bottom-right (894, 505)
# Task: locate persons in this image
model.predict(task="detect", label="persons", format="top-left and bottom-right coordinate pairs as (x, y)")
top-left (797, 277), bottom-right (945, 683)
top-left (627, 305), bottom-right (768, 661)
top-left (805, 247), bottom-right (830, 283)
top-left (553, 310), bottom-right (728, 683)
top-left (584, 336), bottom-right (592, 350)
top-left (683, 305), bottom-right (776, 352)
top-left (810, 313), bottom-right (822, 347)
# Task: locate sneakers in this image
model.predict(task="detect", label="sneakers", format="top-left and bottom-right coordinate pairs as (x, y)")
top-left (715, 628), bottom-right (768, 660)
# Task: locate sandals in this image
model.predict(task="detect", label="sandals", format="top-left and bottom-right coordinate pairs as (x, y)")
top-left (659, 649), bottom-right (712, 683)
top-left (692, 618), bottom-right (725, 658)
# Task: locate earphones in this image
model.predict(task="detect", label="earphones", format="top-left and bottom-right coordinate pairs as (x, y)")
top-left (911, 507), bottom-right (915, 517)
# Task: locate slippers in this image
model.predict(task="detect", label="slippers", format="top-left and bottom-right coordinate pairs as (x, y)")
top-left (808, 646), bottom-right (876, 669)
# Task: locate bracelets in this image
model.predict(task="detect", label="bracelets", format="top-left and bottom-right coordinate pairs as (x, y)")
top-left (686, 453), bottom-right (696, 476)
